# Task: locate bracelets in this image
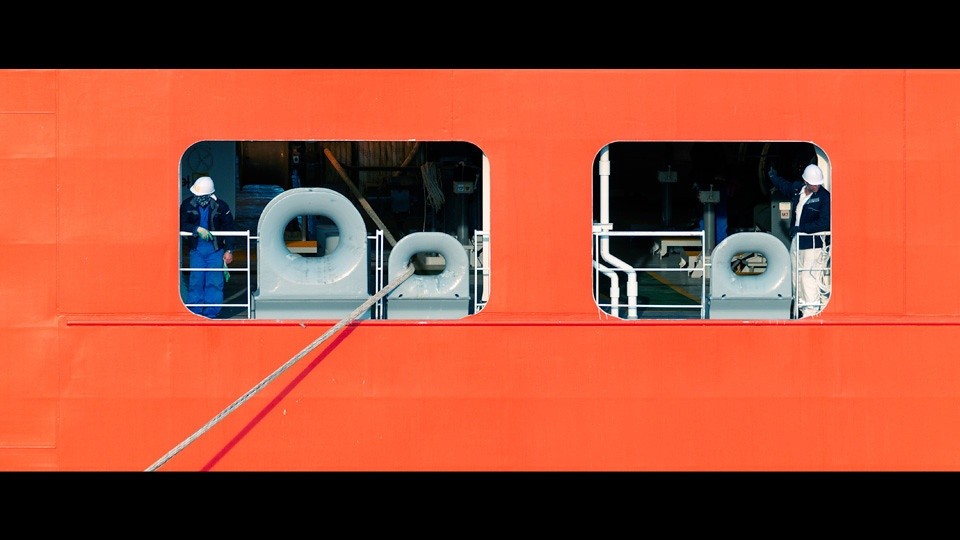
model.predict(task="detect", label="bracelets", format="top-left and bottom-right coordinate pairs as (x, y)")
top-left (225, 249), bottom-right (234, 254)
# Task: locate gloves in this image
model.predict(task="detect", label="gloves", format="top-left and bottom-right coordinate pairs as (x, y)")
top-left (196, 226), bottom-right (213, 242)
top-left (223, 261), bottom-right (231, 282)
top-left (768, 166), bottom-right (778, 180)
top-left (789, 226), bottom-right (801, 238)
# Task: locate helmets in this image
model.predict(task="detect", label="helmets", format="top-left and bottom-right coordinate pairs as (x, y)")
top-left (802, 164), bottom-right (824, 186)
top-left (190, 176), bottom-right (215, 196)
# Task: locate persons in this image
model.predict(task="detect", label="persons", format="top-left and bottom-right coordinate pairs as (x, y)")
top-left (693, 158), bottom-right (733, 243)
top-left (181, 174), bottom-right (235, 319)
top-left (767, 162), bottom-right (831, 319)
top-left (390, 177), bottom-right (412, 236)
